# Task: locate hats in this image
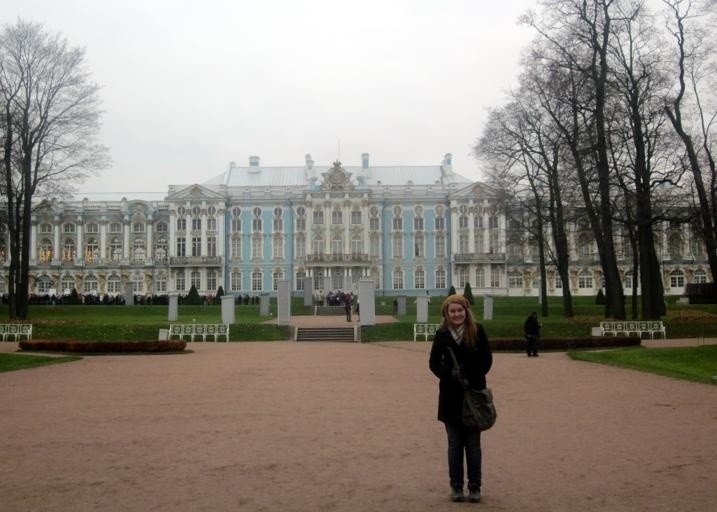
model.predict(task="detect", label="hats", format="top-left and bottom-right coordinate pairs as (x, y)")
top-left (441, 295), bottom-right (470, 317)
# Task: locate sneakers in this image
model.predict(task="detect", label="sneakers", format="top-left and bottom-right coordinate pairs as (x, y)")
top-left (451, 488), bottom-right (464, 501)
top-left (468, 489), bottom-right (481, 502)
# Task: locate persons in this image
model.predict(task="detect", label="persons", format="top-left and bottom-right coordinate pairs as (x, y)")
top-left (521, 312), bottom-right (541, 357)
top-left (316, 288), bottom-right (357, 323)
top-left (427, 293), bottom-right (492, 500)
top-left (390, 299), bottom-right (400, 317)
top-left (32, 294), bottom-right (258, 305)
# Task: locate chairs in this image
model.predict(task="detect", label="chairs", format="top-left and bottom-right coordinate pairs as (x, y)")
top-left (413, 324), bottom-right (441, 342)
top-left (600, 321), bottom-right (667, 339)
top-left (168, 324), bottom-right (230, 342)
top-left (0, 323), bottom-right (33, 341)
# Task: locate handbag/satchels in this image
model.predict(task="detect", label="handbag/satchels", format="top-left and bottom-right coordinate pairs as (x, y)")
top-left (461, 389), bottom-right (496, 430)
